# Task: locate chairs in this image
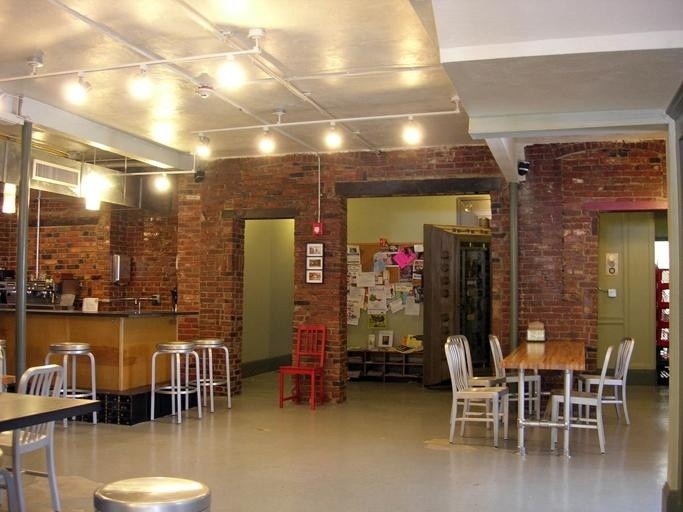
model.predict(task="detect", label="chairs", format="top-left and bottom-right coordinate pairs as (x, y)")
top-left (488, 334), bottom-right (542, 421)
top-left (575, 335), bottom-right (635, 425)
top-left (447, 334), bottom-right (506, 428)
top-left (551, 346), bottom-right (614, 455)
top-left (0, 364), bottom-right (63, 512)
top-left (444, 341), bottom-right (510, 448)
top-left (0, 466), bottom-right (18, 512)
top-left (279, 323), bottom-right (326, 410)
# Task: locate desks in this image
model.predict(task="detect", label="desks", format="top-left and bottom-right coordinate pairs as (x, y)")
top-left (498, 339), bottom-right (586, 457)
top-left (0, 392), bottom-right (100, 433)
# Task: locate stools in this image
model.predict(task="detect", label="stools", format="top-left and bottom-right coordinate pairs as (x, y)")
top-left (93, 475), bottom-right (212, 510)
top-left (185, 337), bottom-right (232, 413)
top-left (45, 341), bottom-right (97, 428)
top-left (0, 340), bottom-right (8, 392)
top-left (150, 341), bottom-right (202, 424)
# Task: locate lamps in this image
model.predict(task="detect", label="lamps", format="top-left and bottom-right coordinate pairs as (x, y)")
top-left (2, 183), bottom-right (17, 214)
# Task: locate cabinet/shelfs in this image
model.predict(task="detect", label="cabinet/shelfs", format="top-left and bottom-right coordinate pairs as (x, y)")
top-left (656, 267), bottom-right (669, 348)
top-left (347, 346), bottom-right (425, 382)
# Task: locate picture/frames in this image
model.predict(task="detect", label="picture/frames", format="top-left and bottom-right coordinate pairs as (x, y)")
top-left (305, 270), bottom-right (324, 284)
top-left (305, 256), bottom-right (324, 270)
top-left (305, 242), bottom-right (324, 257)
